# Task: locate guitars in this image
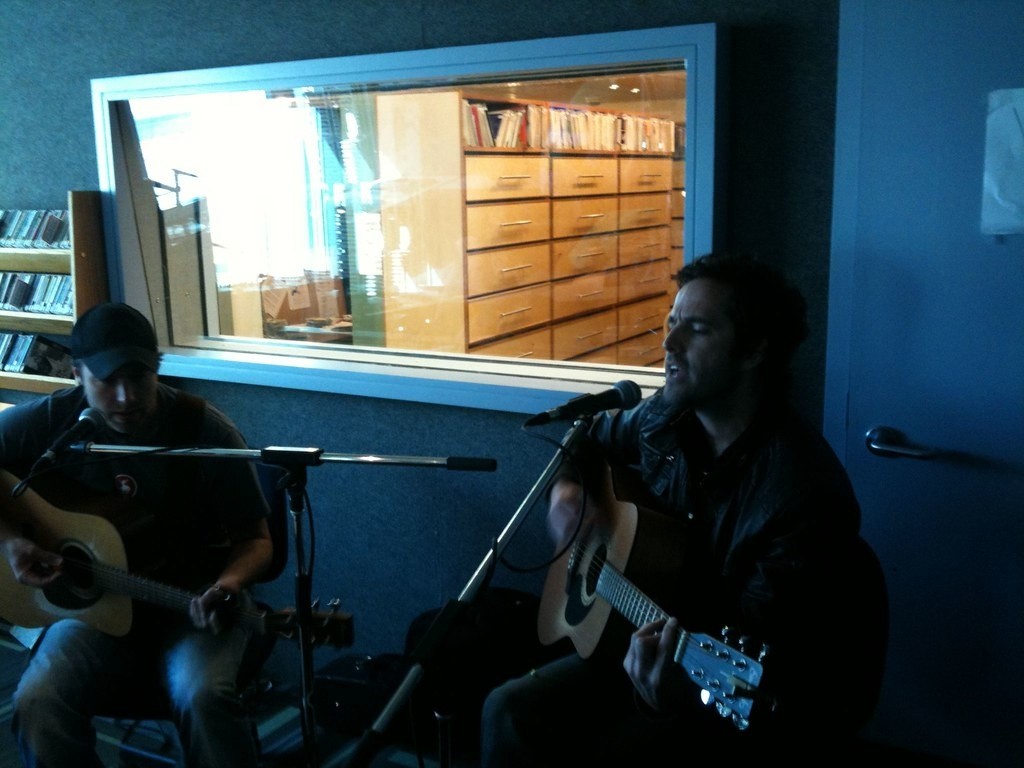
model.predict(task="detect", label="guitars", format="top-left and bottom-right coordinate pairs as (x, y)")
top-left (537, 465), bottom-right (773, 735)
top-left (1, 465), bottom-right (358, 654)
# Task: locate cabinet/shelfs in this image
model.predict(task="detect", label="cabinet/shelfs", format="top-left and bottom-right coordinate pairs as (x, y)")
top-left (377, 92), bottom-right (674, 366)
top-left (1, 191), bottom-right (106, 409)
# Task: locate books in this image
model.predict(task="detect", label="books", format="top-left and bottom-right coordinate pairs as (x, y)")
top-left (463, 102), bottom-right (675, 156)
top-left (0, 208), bottom-right (76, 382)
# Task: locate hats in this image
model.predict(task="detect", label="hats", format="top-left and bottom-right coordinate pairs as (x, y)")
top-left (70, 301), bottom-right (156, 381)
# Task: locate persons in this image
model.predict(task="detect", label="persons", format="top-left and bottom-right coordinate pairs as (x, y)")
top-left (418, 248), bottom-right (866, 768)
top-left (0, 303), bottom-right (272, 767)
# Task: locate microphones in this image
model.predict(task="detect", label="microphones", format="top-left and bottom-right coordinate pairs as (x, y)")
top-left (525, 381), bottom-right (642, 427)
top-left (29, 408), bottom-right (107, 480)
